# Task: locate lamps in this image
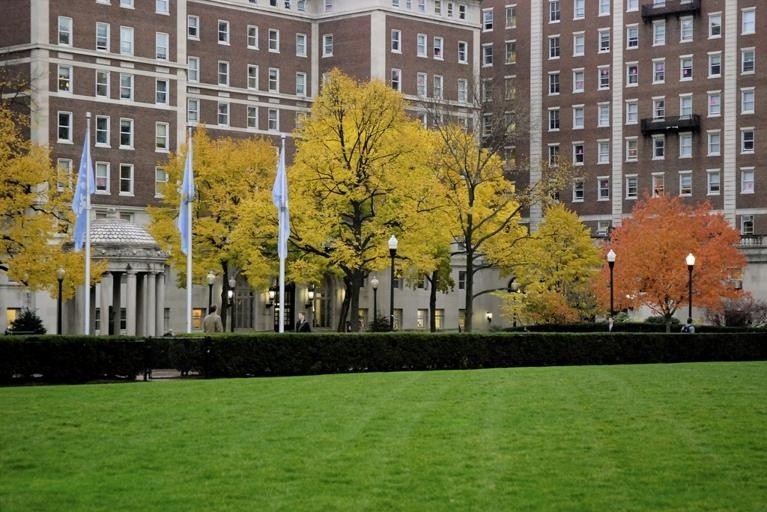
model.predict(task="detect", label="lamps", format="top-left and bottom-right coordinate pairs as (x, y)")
top-left (264, 286), bottom-right (276, 309)
top-left (228, 291), bottom-right (234, 309)
top-left (486, 311), bottom-right (492, 323)
top-left (304, 287), bottom-right (315, 310)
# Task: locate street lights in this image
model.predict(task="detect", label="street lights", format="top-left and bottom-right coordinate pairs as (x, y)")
top-left (606, 248), bottom-right (617, 317)
top-left (205, 271), bottom-right (216, 316)
top-left (685, 253), bottom-right (695, 324)
top-left (371, 275), bottom-right (379, 323)
top-left (55, 264), bottom-right (66, 334)
top-left (387, 234), bottom-right (398, 329)
top-left (229, 276), bottom-right (236, 332)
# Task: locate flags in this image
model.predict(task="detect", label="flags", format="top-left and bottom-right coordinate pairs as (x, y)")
top-left (269, 140), bottom-right (291, 260)
top-left (176, 137), bottom-right (196, 258)
top-left (69, 120), bottom-right (96, 253)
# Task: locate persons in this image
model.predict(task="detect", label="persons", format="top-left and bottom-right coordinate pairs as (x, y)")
top-left (679, 317), bottom-right (695, 334)
top-left (295, 311), bottom-right (312, 332)
top-left (202, 303), bottom-right (224, 333)
top-left (709, 313), bottom-right (720, 326)
